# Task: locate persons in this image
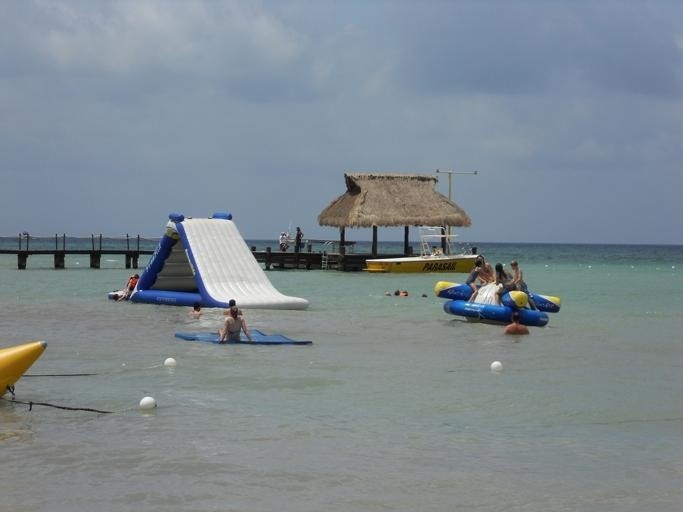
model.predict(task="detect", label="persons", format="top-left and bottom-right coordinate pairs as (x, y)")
top-left (223, 299), bottom-right (242, 316)
top-left (465, 254), bottom-right (540, 311)
top-left (279, 232), bottom-right (290, 251)
top-left (394, 289), bottom-right (409, 296)
top-left (218, 306), bottom-right (251, 341)
top-left (118, 274), bottom-right (139, 301)
top-left (295, 226), bottom-right (304, 253)
top-left (189, 303), bottom-right (201, 313)
top-left (503, 311), bottom-right (530, 334)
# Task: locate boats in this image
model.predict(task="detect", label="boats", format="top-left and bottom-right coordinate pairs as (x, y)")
top-left (362, 226), bottom-right (480, 273)
top-left (434, 281), bottom-right (561, 327)
